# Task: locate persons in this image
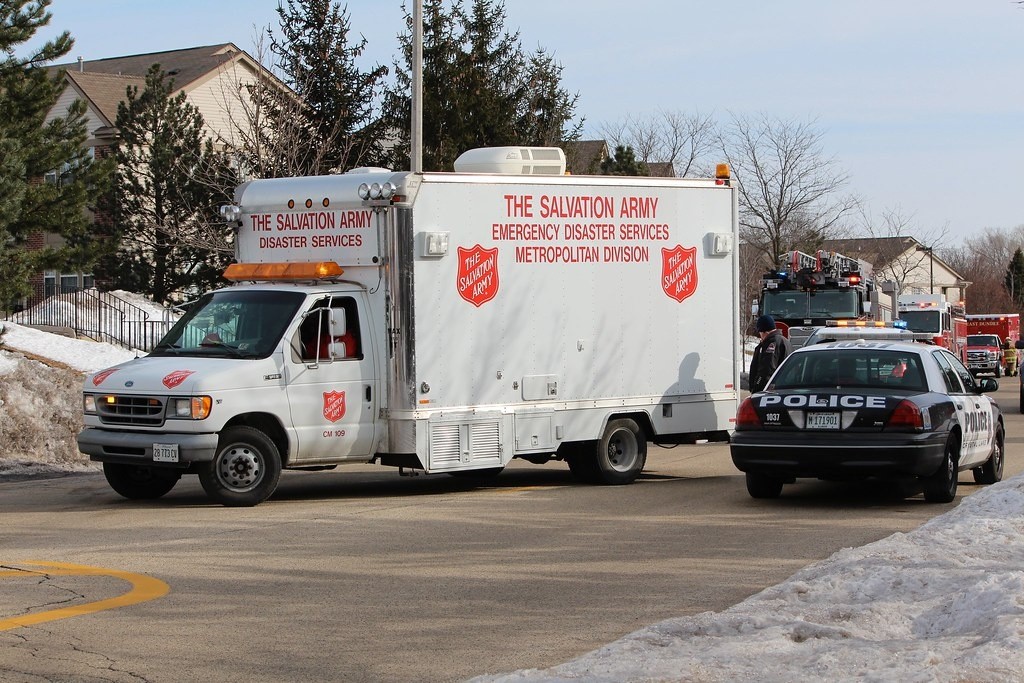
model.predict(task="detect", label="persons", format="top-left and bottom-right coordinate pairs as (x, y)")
top-left (306, 304), bottom-right (357, 357)
top-left (1005, 336), bottom-right (1019, 377)
top-left (749, 314), bottom-right (796, 393)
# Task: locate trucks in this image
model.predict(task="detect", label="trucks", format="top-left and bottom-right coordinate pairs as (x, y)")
top-left (74, 140), bottom-right (742, 510)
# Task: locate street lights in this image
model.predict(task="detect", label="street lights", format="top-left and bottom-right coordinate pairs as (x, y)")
top-left (914, 247), bottom-right (934, 294)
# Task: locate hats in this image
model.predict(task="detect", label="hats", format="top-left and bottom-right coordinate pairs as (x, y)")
top-left (756, 315), bottom-right (776, 332)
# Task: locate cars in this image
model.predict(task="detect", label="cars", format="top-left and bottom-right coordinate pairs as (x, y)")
top-left (728, 338), bottom-right (1008, 504)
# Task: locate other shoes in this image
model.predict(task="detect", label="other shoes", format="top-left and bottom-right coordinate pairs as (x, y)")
top-left (1010, 373), bottom-right (1013, 377)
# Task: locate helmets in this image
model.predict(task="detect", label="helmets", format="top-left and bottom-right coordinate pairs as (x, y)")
top-left (201, 333), bottom-right (221, 349)
top-left (1005, 337), bottom-right (1012, 341)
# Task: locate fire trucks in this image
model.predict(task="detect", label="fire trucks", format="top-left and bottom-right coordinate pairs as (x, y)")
top-left (745, 249), bottom-right (899, 343)
top-left (892, 292), bottom-right (969, 374)
top-left (962, 312), bottom-right (1020, 378)
